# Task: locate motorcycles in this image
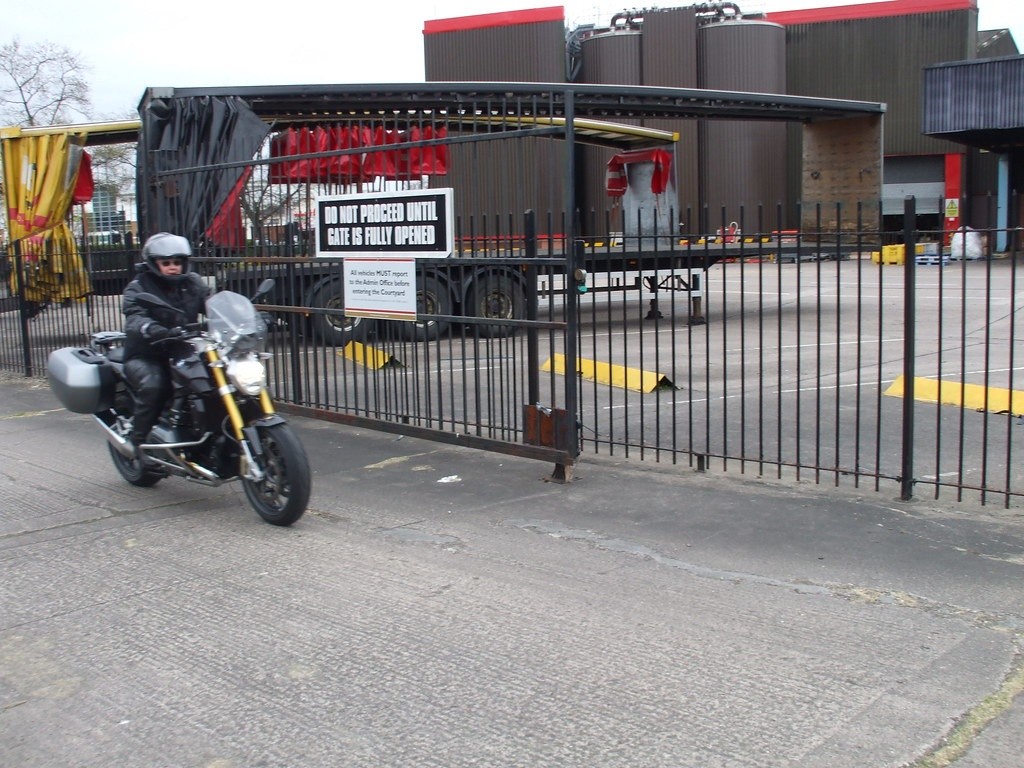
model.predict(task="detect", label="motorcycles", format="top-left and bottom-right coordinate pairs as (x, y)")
top-left (46, 278), bottom-right (312, 527)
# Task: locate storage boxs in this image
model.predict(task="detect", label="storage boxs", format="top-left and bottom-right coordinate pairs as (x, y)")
top-left (872, 242), bottom-right (939, 265)
top-left (47, 346), bottom-right (115, 413)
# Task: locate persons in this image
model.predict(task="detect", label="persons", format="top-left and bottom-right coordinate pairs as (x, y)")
top-left (122, 233), bottom-right (275, 461)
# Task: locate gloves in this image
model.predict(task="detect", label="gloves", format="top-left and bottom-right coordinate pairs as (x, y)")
top-left (166, 327), bottom-right (182, 339)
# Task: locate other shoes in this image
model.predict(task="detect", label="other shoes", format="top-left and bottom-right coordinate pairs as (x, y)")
top-left (129, 429), bottom-right (151, 456)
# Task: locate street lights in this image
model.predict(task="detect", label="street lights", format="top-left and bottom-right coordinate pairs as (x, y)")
top-left (93, 164), bottom-right (104, 244)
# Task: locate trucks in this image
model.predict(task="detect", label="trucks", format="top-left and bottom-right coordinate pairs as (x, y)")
top-left (86, 230), bottom-right (121, 246)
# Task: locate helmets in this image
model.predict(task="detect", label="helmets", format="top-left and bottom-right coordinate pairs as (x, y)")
top-left (141, 232), bottom-right (192, 282)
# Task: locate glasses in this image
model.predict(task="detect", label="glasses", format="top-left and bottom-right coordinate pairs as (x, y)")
top-left (157, 261), bottom-right (182, 266)
